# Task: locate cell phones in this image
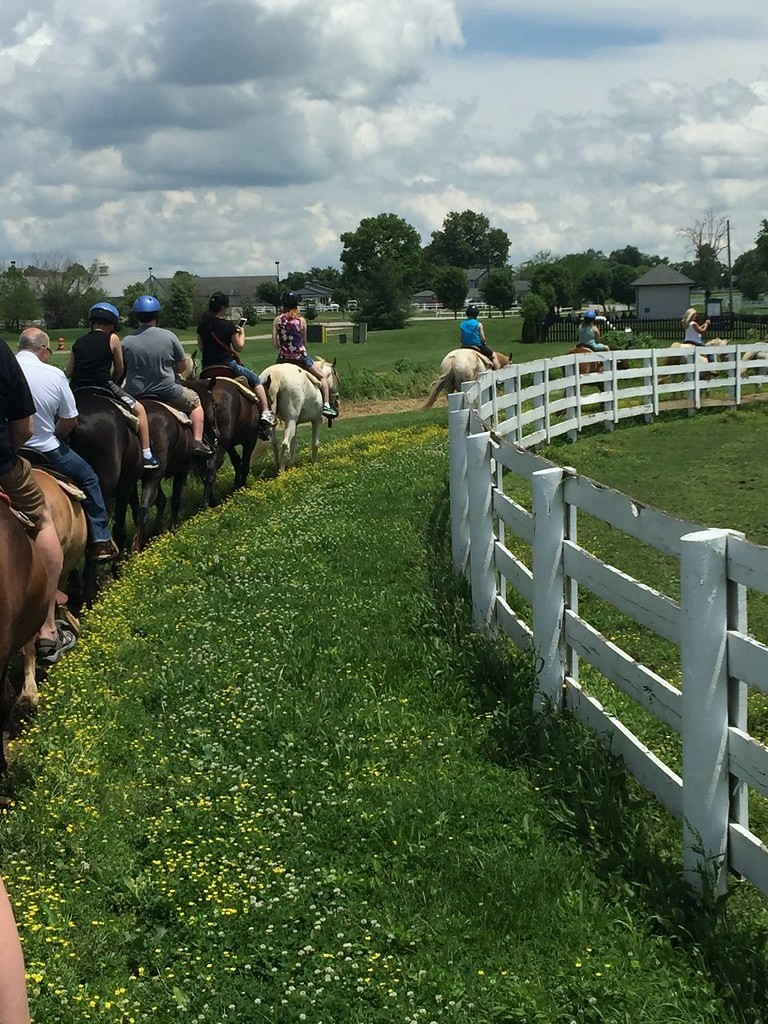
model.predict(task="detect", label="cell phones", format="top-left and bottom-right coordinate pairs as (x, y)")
top-left (236, 318), bottom-right (247, 332)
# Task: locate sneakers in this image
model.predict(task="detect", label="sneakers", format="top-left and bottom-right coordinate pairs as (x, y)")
top-left (144, 456), bottom-right (160, 469)
top-left (261, 411), bottom-right (277, 425)
top-left (92, 542), bottom-right (112, 559)
top-left (194, 441), bottom-right (212, 454)
top-left (322, 406), bottom-right (337, 415)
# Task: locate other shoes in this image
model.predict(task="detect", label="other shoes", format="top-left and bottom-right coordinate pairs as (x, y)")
top-left (708, 371), bottom-right (719, 377)
top-left (497, 381), bottom-right (504, 384)
top-left (34, 630), bottom-right (76, 665)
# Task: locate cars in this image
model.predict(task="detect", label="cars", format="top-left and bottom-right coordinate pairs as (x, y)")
top-left (330, 301), bottom-right (340, 308)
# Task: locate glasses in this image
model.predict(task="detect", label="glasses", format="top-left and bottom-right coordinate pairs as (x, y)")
top-left (43, 344), bottom-right (52, 356)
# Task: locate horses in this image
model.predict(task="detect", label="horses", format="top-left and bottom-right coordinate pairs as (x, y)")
top-left (741, 341), bottom-right (768, 391)
top-left (191, 375), bottom-right (272, 508)
top-left (124, 349), bottom-right (200, 558)
top-left (663, 338), bottom-right (730, 400)
top-left (423, 348), bottom-right (512, 412)
top-left (258, 356), bottom-right (340, 473)
top-left (562, 345), bottom-right (629, 409)
top-left (0, 390), bottom-right (141, 775)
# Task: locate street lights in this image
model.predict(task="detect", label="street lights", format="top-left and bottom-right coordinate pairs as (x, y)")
top-left (11, 261), bottom-right (20, 330)
top-left (149, 268), bottom-right (153, 295)
top-left (275, 262), bottom-right (281, 314)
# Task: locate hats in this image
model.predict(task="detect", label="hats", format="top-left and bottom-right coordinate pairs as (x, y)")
top-left (685, 308), bottom-right (696, 321)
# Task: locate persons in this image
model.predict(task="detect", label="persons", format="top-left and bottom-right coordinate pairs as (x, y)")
top-left (14, 326), bottom-right (120, 564)
top-left (120, 294), bottom-right (213, 455)
top-left (0, 338), bottom-right (77, 666)
top-left (459, 304), bottom-right (500, 369)
top-left (272, 289), bottom-right (339, 418)
top-left (577, 309), bottom-right (610, 351)
top-left (66, 302), bottom-right (161, 469)
top-left (196, 291), bottom-right (277, 427)
top-left (680, 307), bottom-right (720, 376)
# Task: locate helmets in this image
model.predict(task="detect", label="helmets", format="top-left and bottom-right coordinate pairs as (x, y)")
top-left (90, 303), bottom-right (119, 323)
top-left (133, 296), bottom-right (160, 312)
top-left (466, 306), bottom-right (479, 314)
top-left (585, 310), bottom-right (596, 318)
top-left (283, 291), bottom-right (299, 304)
